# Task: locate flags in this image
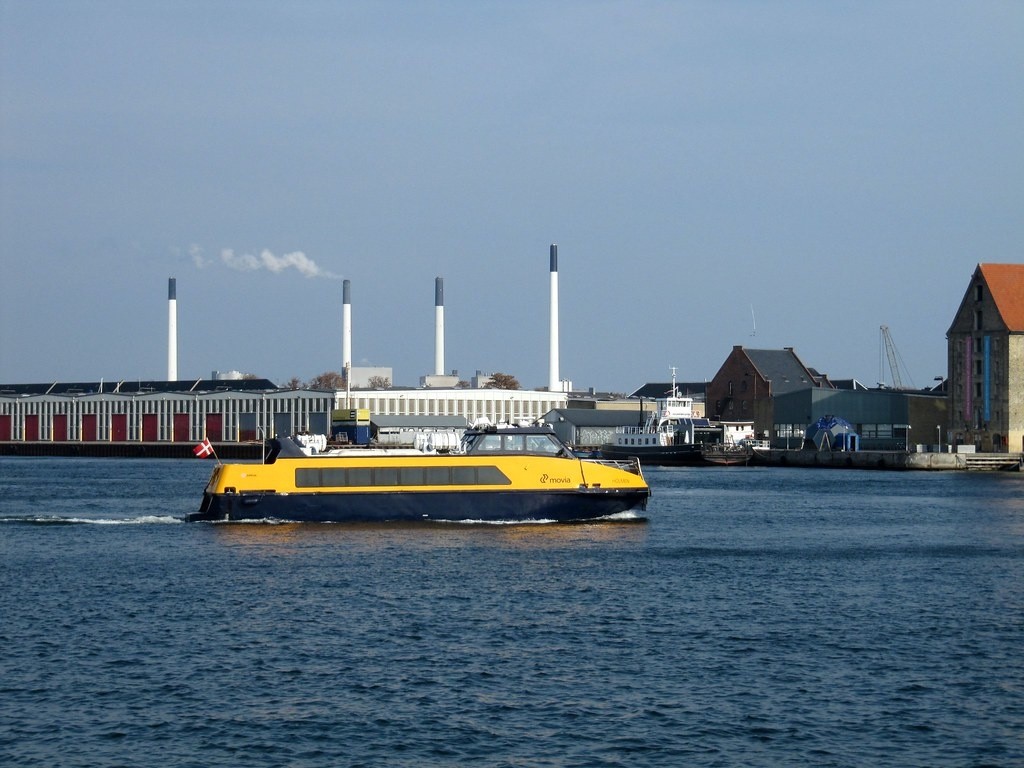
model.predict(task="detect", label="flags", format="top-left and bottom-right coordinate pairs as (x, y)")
top-left (192, 439), bottom-right (213, 459)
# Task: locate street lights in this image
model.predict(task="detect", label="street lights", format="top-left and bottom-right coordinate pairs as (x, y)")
top-left (933, 376), bottom-right (945, 393)
top-left (906, 425), bottom-right (912, 452)
top-left (936, 425), bottom-right (941, 453)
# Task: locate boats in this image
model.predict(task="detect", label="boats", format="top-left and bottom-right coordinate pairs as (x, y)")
top-left (674, 448), bottom-right (754, 466)
top-left (183, 425), bottom-right (652, 524)
top-left (599, 432), bottom-right (704, 464)
